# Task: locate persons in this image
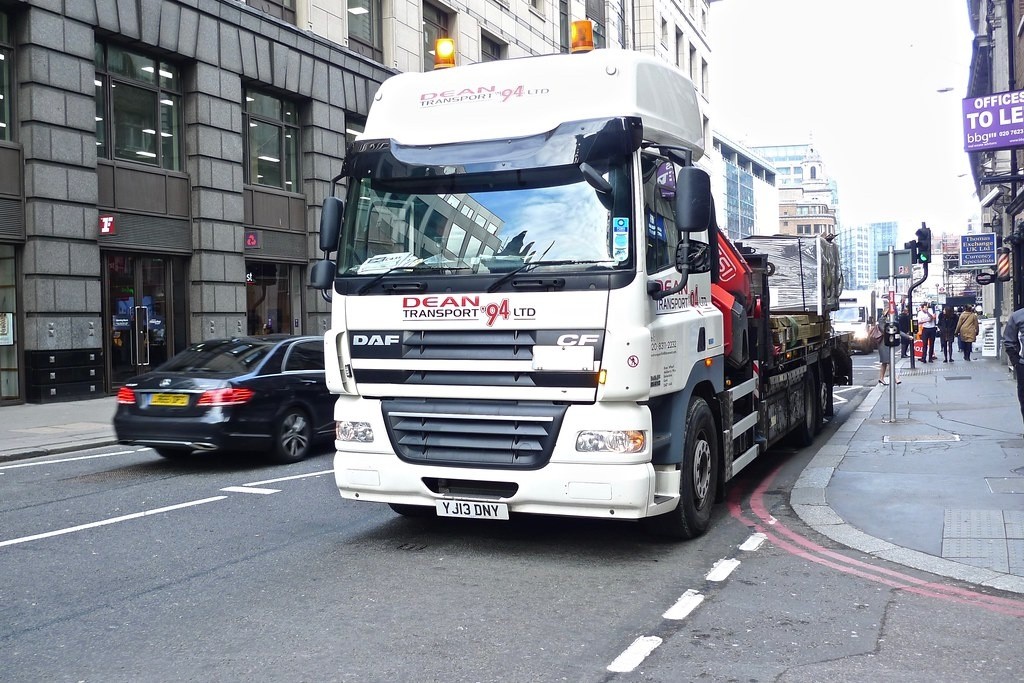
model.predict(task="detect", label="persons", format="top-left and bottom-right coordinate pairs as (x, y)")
top-left (876, 302), bottom-right (979, 386)
top-left (1002, 308), bottom-right (1024, 420)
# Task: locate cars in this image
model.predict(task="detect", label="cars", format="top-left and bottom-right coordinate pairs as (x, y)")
top-left (112, 334), bottom-right (336, 464)
top-left (113, 315), bottom-right (155, 360)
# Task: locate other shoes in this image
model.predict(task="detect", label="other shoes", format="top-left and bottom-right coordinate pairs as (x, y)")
top-left (901, 355), bottom-right (910, 358)
top-left (920, 360), bottom-right (926, 363)
top-left (929, 361), bottom-right (933, 363)
top-left (943, 360), bottom-right (947, 362)
top-left (949, 359), bottom-right (954, 362)
top-left (932, 356), bottom-right (937, 360)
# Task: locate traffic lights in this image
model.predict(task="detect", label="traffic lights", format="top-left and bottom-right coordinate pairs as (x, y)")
top-left (904, 240), bottom-right (917, 264)
top-left (916, 228), bottom-right (931, 263)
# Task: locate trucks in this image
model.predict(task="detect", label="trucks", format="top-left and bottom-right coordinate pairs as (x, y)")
top-left (831, 289), bottom-right (879, 355)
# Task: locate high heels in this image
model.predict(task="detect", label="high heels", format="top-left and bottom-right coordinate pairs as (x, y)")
top-left (878, 380), bottom-right (889, 385)
top-left (897, 382), bottom-right (901, 384)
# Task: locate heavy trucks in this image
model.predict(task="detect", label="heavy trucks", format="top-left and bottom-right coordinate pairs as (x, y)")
top-left (305, 22), bottom-right (858, 539)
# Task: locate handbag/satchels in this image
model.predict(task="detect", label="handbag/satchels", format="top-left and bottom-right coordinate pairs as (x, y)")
top-left (869, 324), bottom-right (883, 344)
top-left (955, 328), bottom-right (960, 337)
